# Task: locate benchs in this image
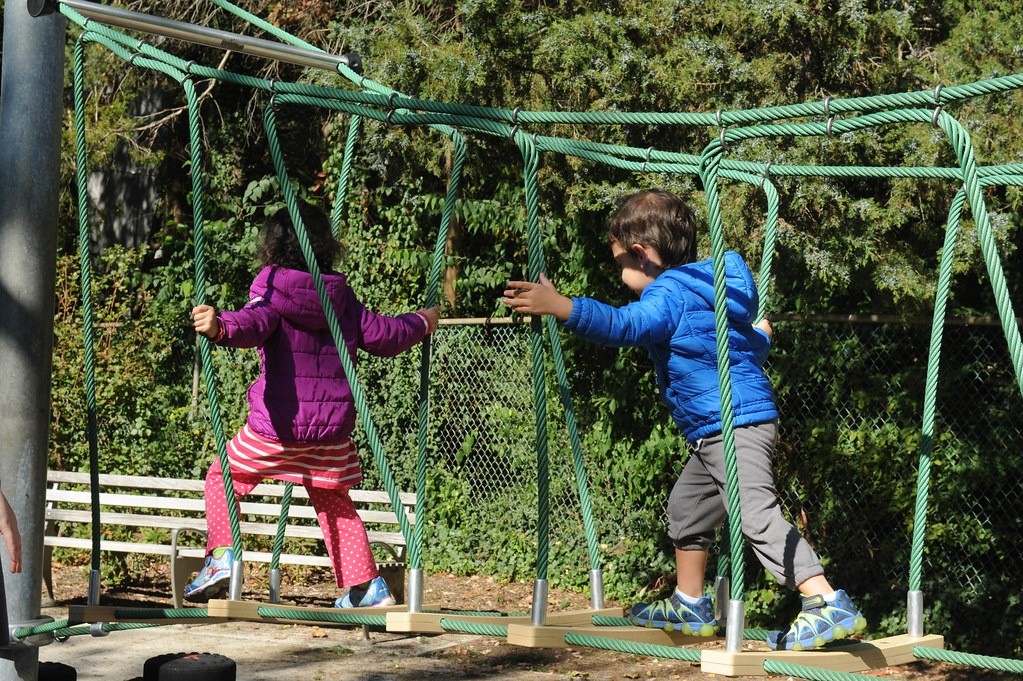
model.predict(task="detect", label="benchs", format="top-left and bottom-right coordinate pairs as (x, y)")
top-left (43, 471), bottom-right (416, 640)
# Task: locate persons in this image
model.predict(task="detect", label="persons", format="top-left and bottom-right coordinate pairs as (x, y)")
top-left (505, 189), bottom-right (868, 652)
top-left (192, 206), bottom-right (442, 608)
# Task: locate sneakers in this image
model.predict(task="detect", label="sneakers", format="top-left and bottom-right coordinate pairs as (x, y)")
top-left (183, 550), bottom-right (243, 602)
top-left (630, 593), bottom-right (720, 638)
top-left (334, 576), bottom-right (396, 610)
top-left (767, 589), bottom-right (867, 651)
